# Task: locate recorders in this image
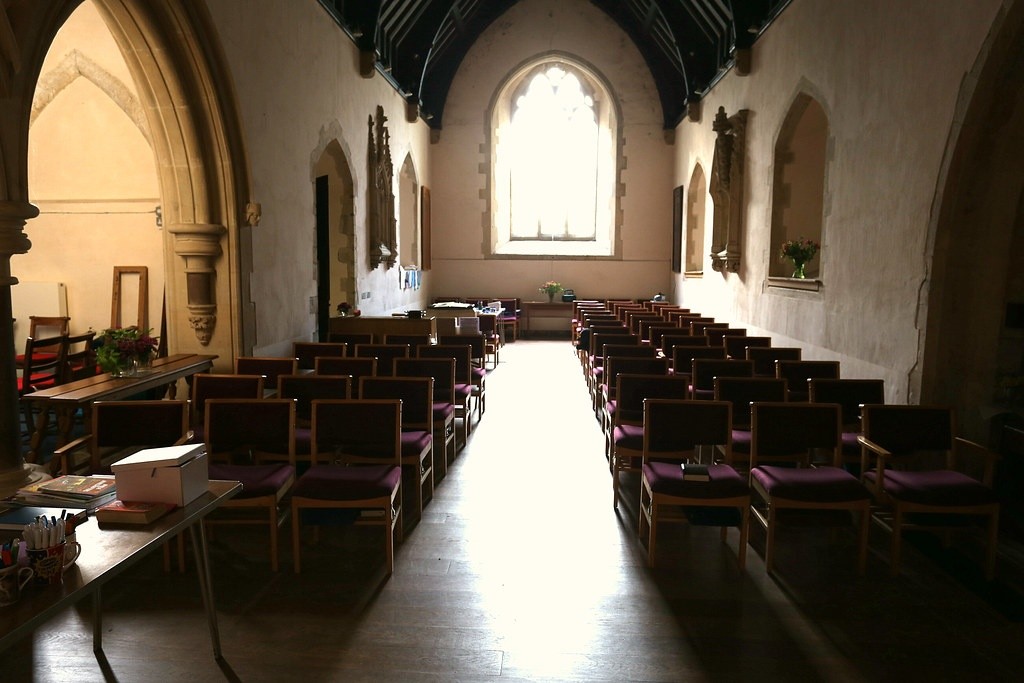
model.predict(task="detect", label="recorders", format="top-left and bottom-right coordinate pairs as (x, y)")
top-left (562, 294), bottom-right (576, 302)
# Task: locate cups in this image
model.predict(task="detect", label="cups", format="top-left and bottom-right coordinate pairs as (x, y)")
top-left (65, 532), bottom-right (76, 565)
top-left (0, 560), bottom-right (34, 608)
top-left (25, 542), bottom-right (81, 587)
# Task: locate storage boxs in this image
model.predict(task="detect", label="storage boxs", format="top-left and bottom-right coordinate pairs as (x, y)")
top-left (111, 443), bottom-right (208, 508)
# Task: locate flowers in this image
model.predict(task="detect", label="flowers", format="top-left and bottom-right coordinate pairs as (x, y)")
top-left (337, 303), bottom-right (352, 313)
top-left (95, 326), bottom-right (161, 373)
top-left (539, 280), bottom-right (563, 298)
top-left (780, 235), bottom-right (820, 273)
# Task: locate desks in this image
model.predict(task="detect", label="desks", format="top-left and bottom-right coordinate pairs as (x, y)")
top-left (522, 300), bottom-right (573, 339)
top-left (1, 475), bottom-right (243, 660)
top-left (329, 316), bottom-right (437, 337)
top-left (22, 353), bottom-right (217, 463)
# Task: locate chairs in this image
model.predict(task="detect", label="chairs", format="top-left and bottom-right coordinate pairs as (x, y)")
top-left (572, 299), bottom-right (1000, 585)
top-left (15, 296), bottom-right (521, 577)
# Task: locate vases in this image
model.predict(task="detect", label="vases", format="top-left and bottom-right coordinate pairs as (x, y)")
top-left (789, 257), bottom-right (809, 280)
top-left (547, 291), bottom-right (555, 302)
top-left (110, 352), bottom-right (155, 379)
top-left (341, 311), bottom-right (348, 316)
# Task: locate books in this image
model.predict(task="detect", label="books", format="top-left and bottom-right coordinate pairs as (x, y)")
top-left (95, 499), bottom-right (170, 525)
top-left (0, 474), bottom-right (116, 515)
top-left (0, 506), bottom-right (88, 532)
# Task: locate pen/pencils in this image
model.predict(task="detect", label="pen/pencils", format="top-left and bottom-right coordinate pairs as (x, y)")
top-left (0, 538), bottom-right (20, 569)
top-left (22, 509), bottom-right (66, 549)
